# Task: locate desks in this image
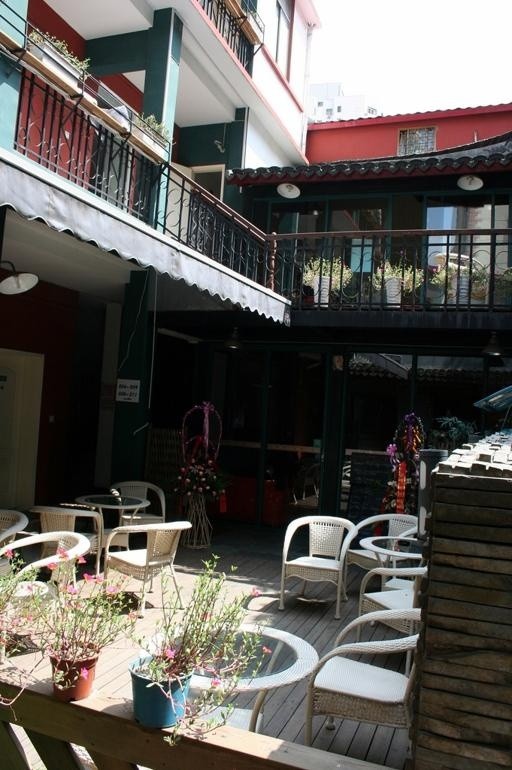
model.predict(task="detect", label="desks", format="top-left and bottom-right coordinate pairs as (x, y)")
top-left (145, 622), bottom-right (320, 737)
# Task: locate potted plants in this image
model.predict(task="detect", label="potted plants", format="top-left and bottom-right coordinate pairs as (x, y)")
top-left (124, 109), bottom-right (176, 163)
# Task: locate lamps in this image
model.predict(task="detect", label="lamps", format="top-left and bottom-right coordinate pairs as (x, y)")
top-left (483, 332), bottom-right (504, 357)
top-left (275, 183), bottom-right (304, 201)
top-left (455, 176), bottom-right (485, 194)
top-left (0, 260), bottom-right (39, 297)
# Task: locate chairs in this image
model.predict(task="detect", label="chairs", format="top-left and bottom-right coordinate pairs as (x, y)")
top-left (432, 252), bottom-right (493, 310)
top-left (291, 456), bottom-right (321, 506)
top-left (279, 503), bottom-right (430, 755)
top-left (0, 476), bottom-right (192, 666)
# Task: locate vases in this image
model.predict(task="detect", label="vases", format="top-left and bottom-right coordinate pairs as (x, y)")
top-left (47, 642), bottom-right (101, 702)
top-left (312, 275), bottom-right (331, 309)
top-left (128, 658), bottom-right (194, 729)
top-left (29, 43), bottom-right (83, 92)
top-left (385, 278), bottom-right (402, 309)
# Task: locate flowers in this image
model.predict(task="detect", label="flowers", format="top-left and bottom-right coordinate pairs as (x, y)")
top-left (176, 454), bottom-right (228, 504)
top-left (428, 264), bottom-right (488, 296)
top-left (378, 411), bottom-right (428, 533)
top-left (301, 255), bottom-right (355, 292)
top-left (27, 28), bottom-right (90, 75)
top-left (29, 545), bottom-right (139, 688)
top-left (1, 544), bottom-right (69, 724)
top-left (117, 549), bottom-right (272, 745)
top-left (365, 249), bottom-right (425, 295)
